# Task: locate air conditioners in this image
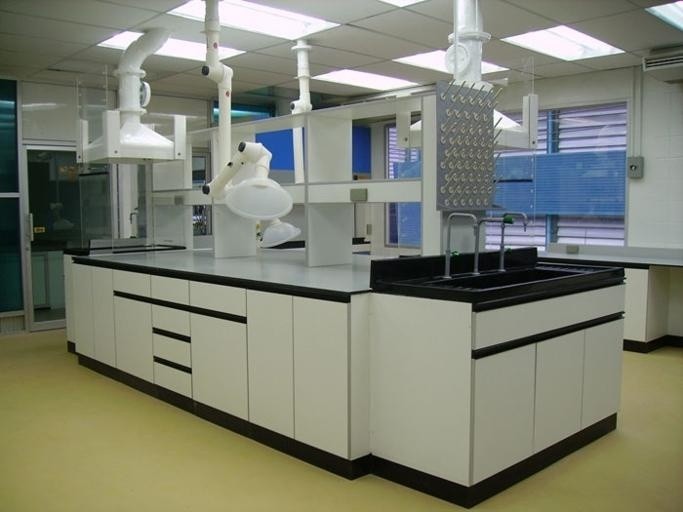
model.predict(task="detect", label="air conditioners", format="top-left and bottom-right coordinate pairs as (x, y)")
top-left (642, 52), bottom-right (683, 81)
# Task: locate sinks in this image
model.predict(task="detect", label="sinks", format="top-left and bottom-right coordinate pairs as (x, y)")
top-left (421, 266), bottom-right (587, 290)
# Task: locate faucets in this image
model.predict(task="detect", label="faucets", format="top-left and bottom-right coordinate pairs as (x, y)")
top-left (444, 212), bottom-right (479, 279)
top-left (499, 211), bottom-right (529, 271)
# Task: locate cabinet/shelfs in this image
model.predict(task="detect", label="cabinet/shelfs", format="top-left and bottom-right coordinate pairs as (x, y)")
top-left (145, 92), bottom-right (486, 268)
top-left (472, 284), bottom-right (626, 507)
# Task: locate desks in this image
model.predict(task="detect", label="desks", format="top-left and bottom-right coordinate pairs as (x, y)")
top-left (537, 243), bottom-right (683, 353)
